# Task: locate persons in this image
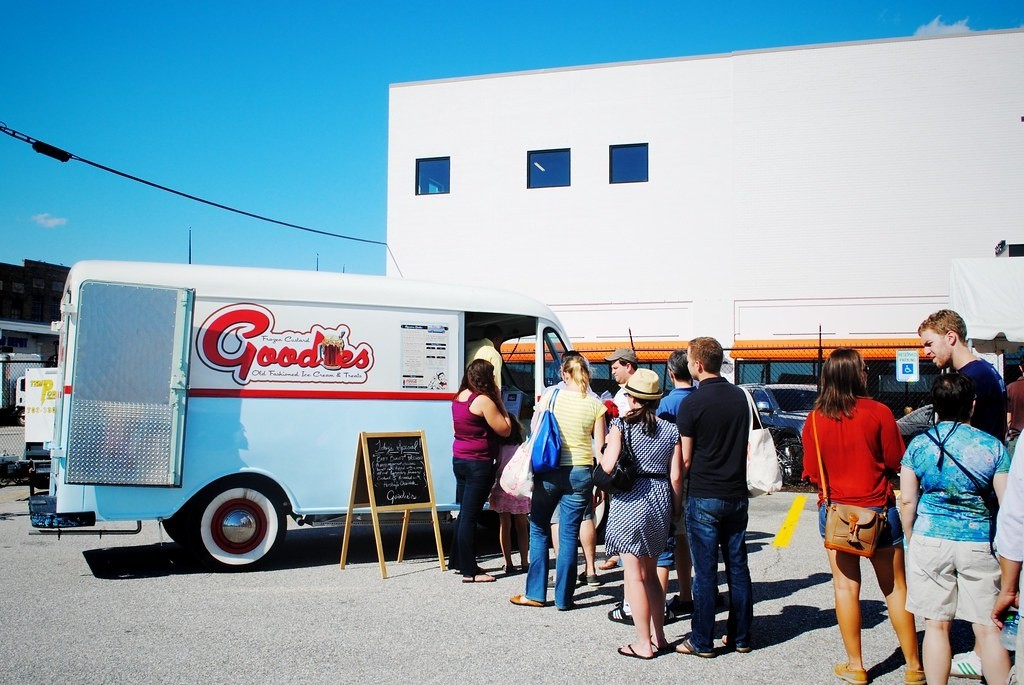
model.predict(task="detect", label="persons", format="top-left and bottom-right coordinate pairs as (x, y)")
top-left (671, 337), bottom-right (753, 657)
top-left (597, 368), bottom-right (682, 658)
top-left (656, 353), bottom-right (697, 610)
top-left (899, 373), bottom-right (1010, 685)
top-left (452, 325), bottom-right (532, 583)
top-left (917, 309), bottom-right (1008, 679)
top-left (991, 352), bottom-right (1024, 685)
top-left (802, 350), bottom-right (926, 685)
top-left (510, 349), bottom-right (638, 612)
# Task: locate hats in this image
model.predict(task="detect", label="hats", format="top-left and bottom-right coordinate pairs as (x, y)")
top-left (604, 347), bottom-right (638, 364)
top-left (617, 367), bottom-right (664, 400)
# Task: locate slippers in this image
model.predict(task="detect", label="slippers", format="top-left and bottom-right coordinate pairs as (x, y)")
top-left (673, 637), bottom-right (715, 658)
top-left (650, 635), bottom-right (670, 651)
top-left (618, 645), bottom-right (654, 659)
top-left (722, 634), bottom-right (751, 653)
top-left (462, 574), bottom-right (495, 583)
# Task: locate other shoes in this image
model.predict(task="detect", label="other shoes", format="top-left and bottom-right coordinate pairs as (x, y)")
top-left (511, 596), bottom-right (546, 606)
top-left (599, 560), bottom-right (619, 570)
top-left (834, 663), bottom-right (868, 685)
top-left (715, 594), bottom-right (724, 608)
top-left (904, 669), bottom-right (926, 685)
top-left (576, 571), bottom-right (600, 586)
top-left (546, 576), bottom-right (556, 589)
top-left (666, 595), bottom-right (694, 613)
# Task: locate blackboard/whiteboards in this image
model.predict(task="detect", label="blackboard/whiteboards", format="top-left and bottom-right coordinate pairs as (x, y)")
top-left (349, 430), bottom-right (435, 512)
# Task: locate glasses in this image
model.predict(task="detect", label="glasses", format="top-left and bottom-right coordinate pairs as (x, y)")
top-left (623, 393), bottom-right (630, 397)
top-left (859, 365), bottom-right (870, 374)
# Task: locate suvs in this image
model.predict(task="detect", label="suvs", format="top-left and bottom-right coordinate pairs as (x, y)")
top-left (735, 383), bottom-right (939, 485)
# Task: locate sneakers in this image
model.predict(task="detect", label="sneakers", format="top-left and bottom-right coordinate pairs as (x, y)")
top-left (607, 600), bottom-right (634, 625)
top-left (954, 653), bottom-right (969, 659)
top-left (950, 651), bottom-right (984, 680)
top-left (664, 603), bottom-right (676, 625)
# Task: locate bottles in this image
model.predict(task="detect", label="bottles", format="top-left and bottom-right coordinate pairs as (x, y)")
top-left (998, 606), bottom-right (1020, 651)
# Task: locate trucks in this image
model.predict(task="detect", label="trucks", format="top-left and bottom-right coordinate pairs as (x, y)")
top-left (0, 260), bottom-right (572, 573)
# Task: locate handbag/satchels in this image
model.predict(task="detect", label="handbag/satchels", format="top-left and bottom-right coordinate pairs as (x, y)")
top-left (592, 418), bottom-right (636, 493)
top-left (532, 388), bottom-right (562, 475)
top-left (738, 384), bottom-right (783, 498)
top-left (985, 490), bottom-right (999, 555)
top-left (499, 438), bottom-right (534, 501)
top-left (824, 503), bottom-right (886, 557)
